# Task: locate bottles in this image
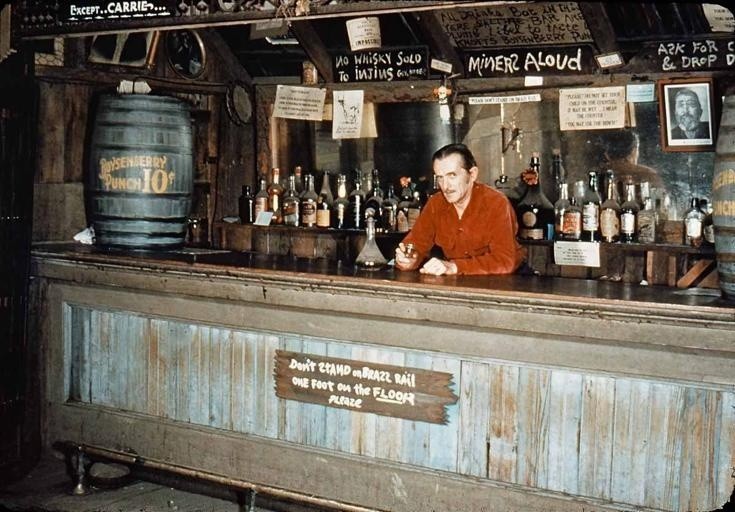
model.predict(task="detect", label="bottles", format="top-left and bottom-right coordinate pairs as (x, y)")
top-left (238, 151), bottom-right (716, 247)
top-left (353, 217), bottom-right (388, 272)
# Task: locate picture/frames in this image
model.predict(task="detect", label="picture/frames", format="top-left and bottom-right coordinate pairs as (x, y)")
top-left (654, 74), bottom-right (719, 155)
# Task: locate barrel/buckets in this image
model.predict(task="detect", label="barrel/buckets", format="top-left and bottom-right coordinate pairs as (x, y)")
top-left (711, 85), bottom-right (735, 300)
top-left (346, 16), bottom-right (382, 52)
top-left (83, 92), bottom-right (197, 253)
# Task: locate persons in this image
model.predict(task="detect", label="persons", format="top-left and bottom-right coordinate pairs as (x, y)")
top-left (395, 144), bottom-right (524, 276)
top-left (597, 128), bottom-right (665, 191)
top-left (672, 90), bottom-right (710, 139)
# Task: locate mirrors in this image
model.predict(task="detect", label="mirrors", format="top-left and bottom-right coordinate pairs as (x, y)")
top-left (267, 85), bottom-right (717, 235)
